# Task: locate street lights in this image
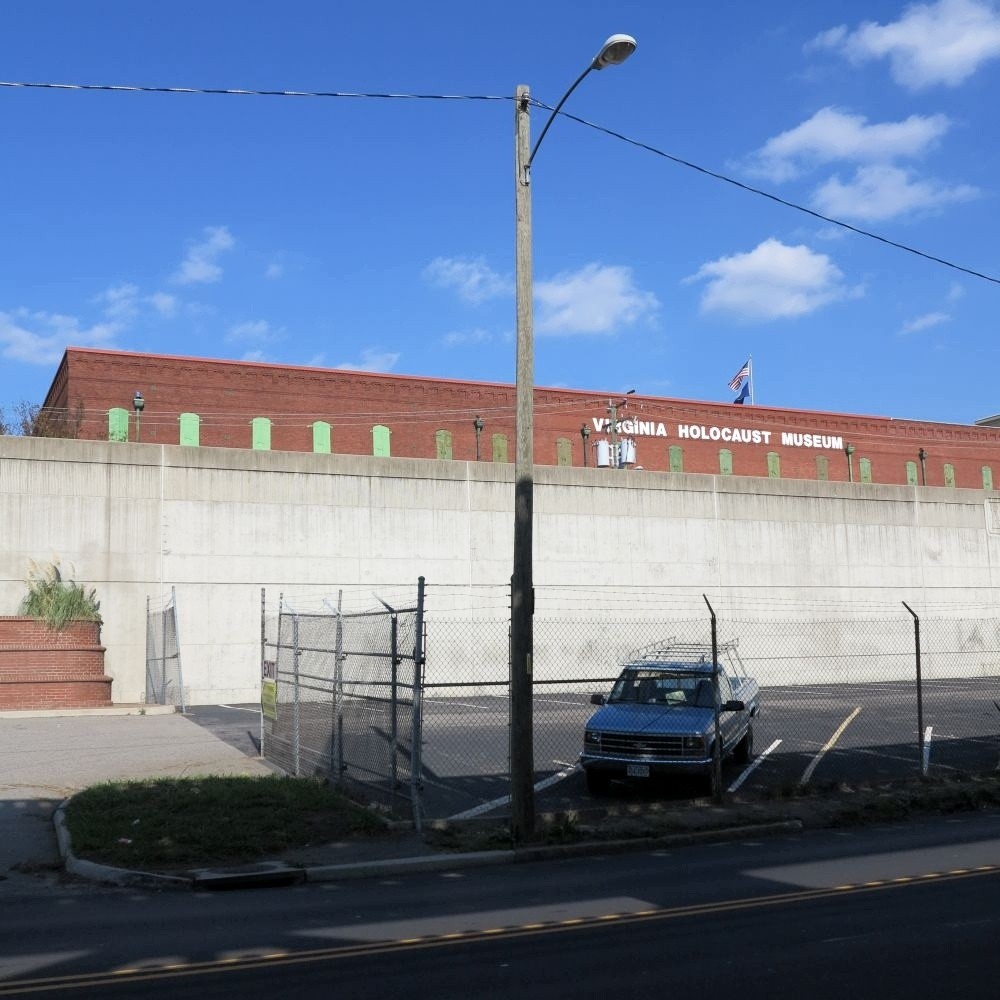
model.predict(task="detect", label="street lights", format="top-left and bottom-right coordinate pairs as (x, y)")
top-left (581, 424), bottom-right (592, 467)
top-left (132, 391), bottom-right (144, 441)
top-left (845, 442), bottom-right (854, 482)
top-left (473, 415), bottom-right (484, 461)
top-left (508, 34), bottom-right (636, 839)
top-left (919, 448), bottom-right (928, 486)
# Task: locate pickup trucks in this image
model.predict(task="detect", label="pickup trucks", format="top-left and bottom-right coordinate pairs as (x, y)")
top-left (580, 636), bottom-right (761, 804)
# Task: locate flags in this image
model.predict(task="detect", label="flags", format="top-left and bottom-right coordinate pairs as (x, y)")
top-left (729, 361), bottom-right (750, 391)
top-left (734, 382), bottom-right (749, 404)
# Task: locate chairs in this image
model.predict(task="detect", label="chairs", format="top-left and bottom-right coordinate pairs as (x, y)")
top-left (637, 679), bottom-right (657, 703)
top-left (692, 679), bottom-right (713, 708)
